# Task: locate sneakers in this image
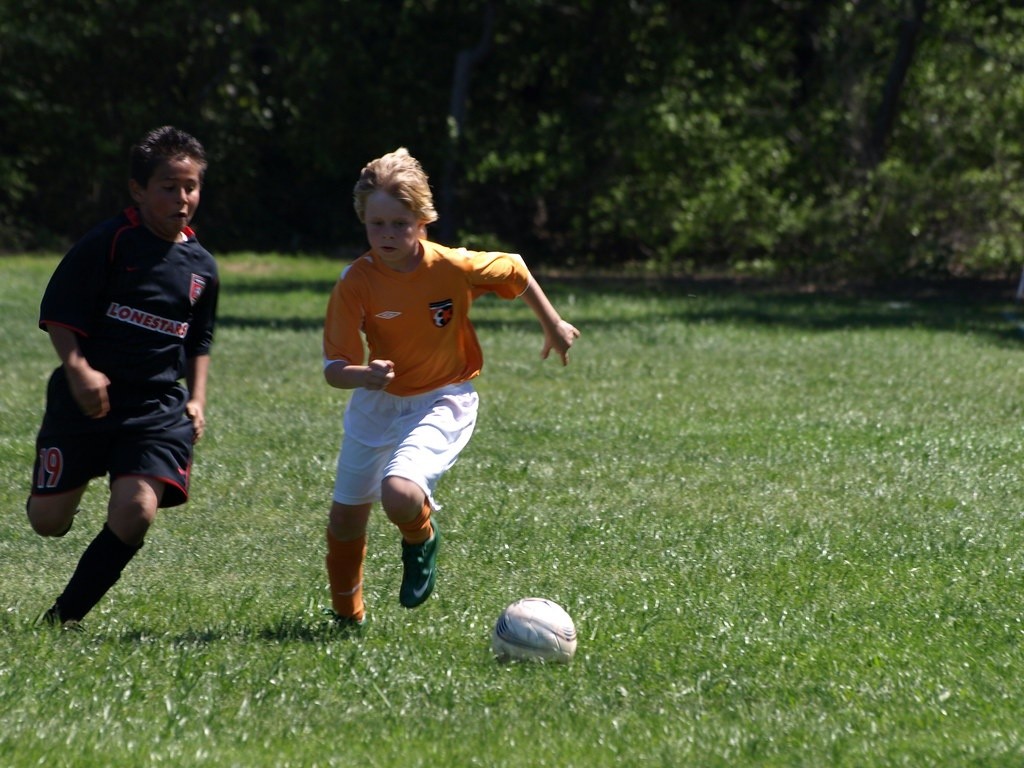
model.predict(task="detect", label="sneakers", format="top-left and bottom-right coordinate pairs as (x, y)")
top-left (311, 609), bottom-right (369, 645)
top-left (400, 516), bottom-right (440, 608)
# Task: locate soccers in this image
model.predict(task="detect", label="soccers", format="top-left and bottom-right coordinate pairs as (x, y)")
top-left (493, 598), bottom-right (579, 667)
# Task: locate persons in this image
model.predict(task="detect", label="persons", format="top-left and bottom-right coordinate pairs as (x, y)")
top-left (26, 125), bottom-right (222, 634)
top-left (323, 146), bottom-right (579, 640)
